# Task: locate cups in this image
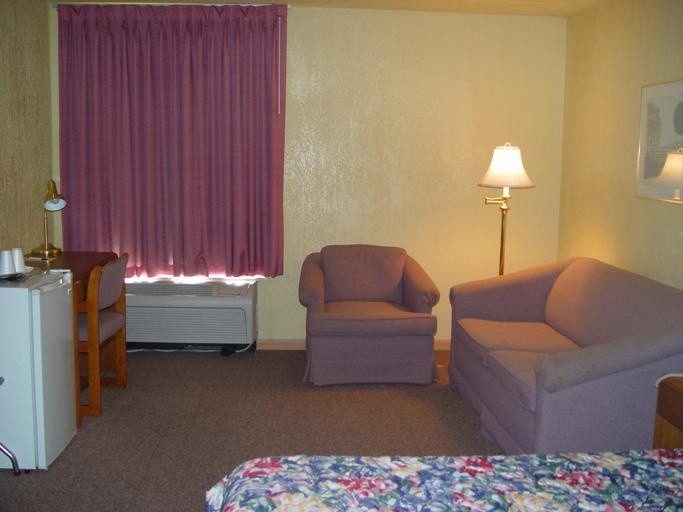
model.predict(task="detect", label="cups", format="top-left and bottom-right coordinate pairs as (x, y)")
top-left (1, 247), bottom-right (27, 275)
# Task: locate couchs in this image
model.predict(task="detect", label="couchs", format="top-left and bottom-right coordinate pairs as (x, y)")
top-left (446, 256), bottom-right (681, 454)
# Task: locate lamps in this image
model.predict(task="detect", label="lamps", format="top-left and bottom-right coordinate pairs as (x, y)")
top-left (477, 142), bottom-right (533, 275)
top-left (33, 179), bottom-right (69, 258)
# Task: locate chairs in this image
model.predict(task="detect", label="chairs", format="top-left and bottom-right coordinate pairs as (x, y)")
top-left (296, 242), bottom-right (440, 386)
top-left (73, 254), bottom-right (130, 425)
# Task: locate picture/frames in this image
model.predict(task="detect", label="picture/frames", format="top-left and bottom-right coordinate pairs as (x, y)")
top-left (634, 81), bottom-right (682, 207)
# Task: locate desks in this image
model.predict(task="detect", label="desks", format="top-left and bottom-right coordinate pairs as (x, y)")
top-left (29, 251), bottom-right (125, 371)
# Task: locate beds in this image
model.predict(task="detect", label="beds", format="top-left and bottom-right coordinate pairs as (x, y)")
top-left (207, 447), bottom-right (683, 512)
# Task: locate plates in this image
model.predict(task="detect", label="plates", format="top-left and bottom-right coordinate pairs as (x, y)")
top-left (1, 267), bottom-right (35, 279)
